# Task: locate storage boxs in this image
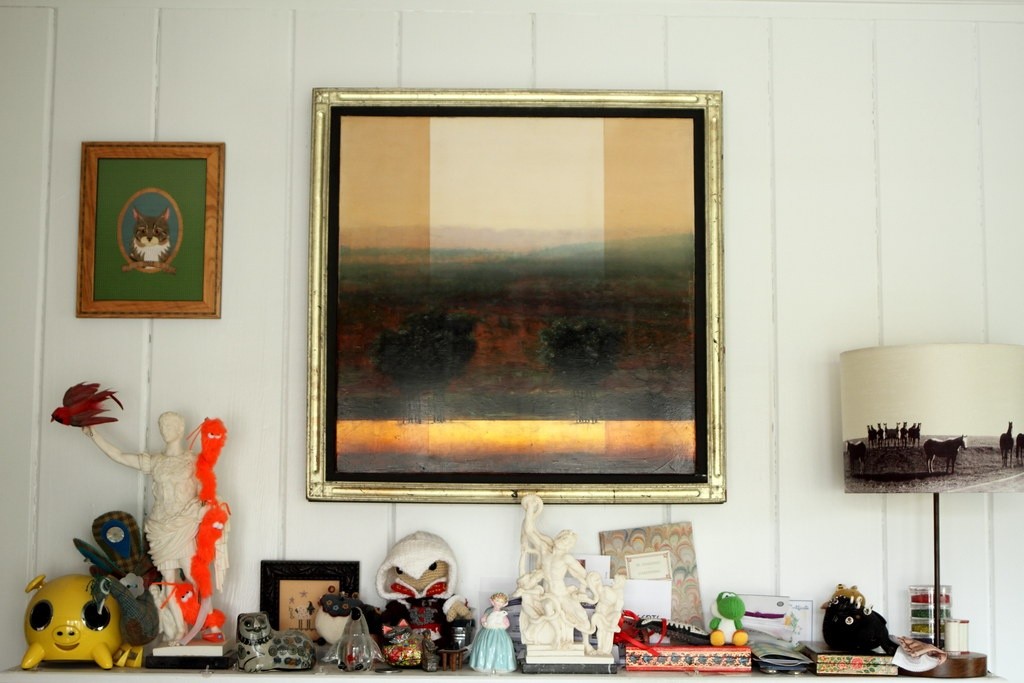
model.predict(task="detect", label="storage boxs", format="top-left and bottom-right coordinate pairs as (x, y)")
top-left (625, 643), bottom-right (752, 672)
top-left (798, 641), bottom-right (898, 675)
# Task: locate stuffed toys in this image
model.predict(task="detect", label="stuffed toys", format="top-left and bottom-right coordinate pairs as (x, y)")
top-left (376, 532), bottom-right (472, 650)
top-left (710, 592), bottom-right (749, 646)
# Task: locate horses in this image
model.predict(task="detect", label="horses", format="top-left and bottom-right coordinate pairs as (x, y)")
top-left (845, 420), bottom-right (1024, 476)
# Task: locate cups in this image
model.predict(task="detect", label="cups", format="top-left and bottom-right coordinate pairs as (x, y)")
top-left (944, 620), bottom-right (969, 653)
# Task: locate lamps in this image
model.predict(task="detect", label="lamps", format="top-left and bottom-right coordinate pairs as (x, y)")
top-left (841, 342), bottom-right (1024, 678)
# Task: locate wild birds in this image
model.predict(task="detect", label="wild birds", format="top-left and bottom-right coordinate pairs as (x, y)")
top-left (50, 381), bottom-right (123, 437)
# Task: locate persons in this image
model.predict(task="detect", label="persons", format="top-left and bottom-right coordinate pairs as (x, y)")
top-left (82, 410), bottom-right (212, 644)
top-left (512, 495), bottom-right (624, 656)
top-left (468, 592), bottom-right (516, 674)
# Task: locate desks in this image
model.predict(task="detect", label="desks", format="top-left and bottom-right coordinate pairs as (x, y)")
top-left (439, 649), bottom-right (468, 670)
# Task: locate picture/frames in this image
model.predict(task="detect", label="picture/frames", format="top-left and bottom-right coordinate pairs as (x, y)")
top-left (75, 141), bottom-right (227, 320)
top-left (304, 88), bottom-right (727, 504)
top-left (260, 560), bottom-right (359, 646)
